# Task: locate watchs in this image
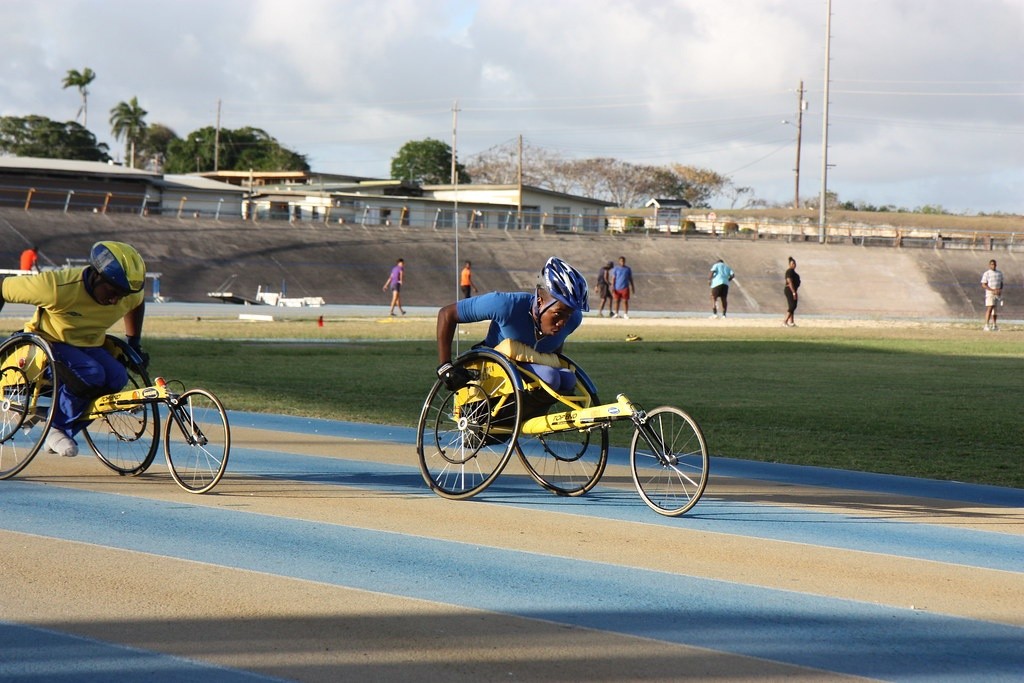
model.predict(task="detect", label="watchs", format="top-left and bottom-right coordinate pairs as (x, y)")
top-left (793, 293), bottom-right (796, 296)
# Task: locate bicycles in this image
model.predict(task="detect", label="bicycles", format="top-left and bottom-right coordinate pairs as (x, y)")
top-left (0, 328), bottom-right (231, 494)
top-left (416, 345), bottom-right (709, 517)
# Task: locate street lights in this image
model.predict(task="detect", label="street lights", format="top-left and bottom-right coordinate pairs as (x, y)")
top-left (780, 81), bottom-right (808, 209)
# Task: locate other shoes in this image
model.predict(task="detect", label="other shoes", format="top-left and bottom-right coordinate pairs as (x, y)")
top-left (402, 311), bottom-right (406, 315)
top-left (789, 323), bottom-right (795, 326)
top-left (983, 324), bottom-right (990, 332)
top-left (612, 313), bottom-right (621, 318)
top-left (389, 312), bottom-right (397, 316)
top-left (713, 314), bottom-right (718, 319)
top-left (597, 312), bottom-right (604, 318)
top-left (721, 315), bottom-right (726, 320)
top-left (607, 313), bottom-right (613, 317)
top-left (783, 323), bottom-right (789, 327)
top-left (623, 314), bottom-right (629, 320)
top-left (992, 326), bottom-right (999, 331)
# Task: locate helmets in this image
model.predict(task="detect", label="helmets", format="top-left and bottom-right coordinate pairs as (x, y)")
top-left (91, 242), bottom-right (145, 297)
top-left (539, 256), bottom-right (589, 317)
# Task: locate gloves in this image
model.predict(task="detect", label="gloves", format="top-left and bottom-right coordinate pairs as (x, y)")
top-left (123, 335), bottom-right (151, 374)
top-left (436, 362), bottom-right (473, 391)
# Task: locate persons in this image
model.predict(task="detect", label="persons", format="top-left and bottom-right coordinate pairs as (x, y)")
top-left (595, 261), bottom-right (615, 318)
top-left (383, 258), bottom-right (406, 316)
top-left (436, 256), bottom-right (589, 445)
top-left (782, 256), bottom-right (801, 327)
top-left (981, 259), bottom-right (1003, 332)
top-left (708, 259), bottom-right (735, 319)
top-left (611, 256), bottom-right (635, 320)
top-left (0, 240), bottom-right (150, 458)
top-left (460, 260), bottom-right (478, 299)
top-left (19, 246), bottom-right (41, 273)
